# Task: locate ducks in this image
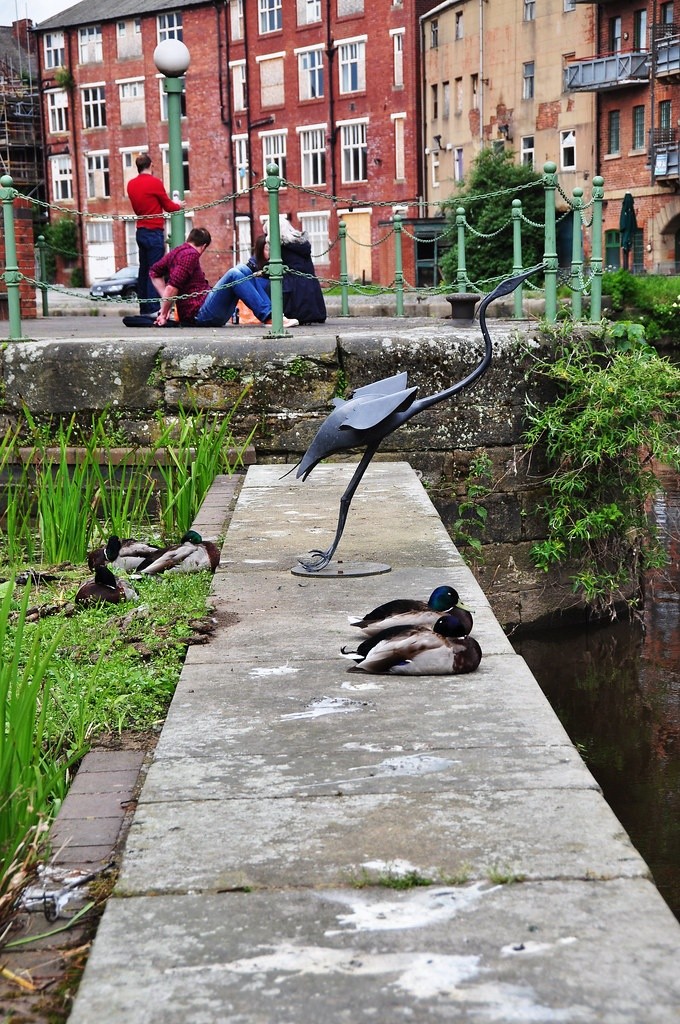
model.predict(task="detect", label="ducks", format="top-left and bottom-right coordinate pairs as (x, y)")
top-left (339, 586), bottom-right (482, 676)
top-left (75, 530), bottom-right (221, 605)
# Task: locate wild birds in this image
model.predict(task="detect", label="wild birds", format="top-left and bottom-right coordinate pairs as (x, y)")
top-left (276, 263), bottom-right (550, 572)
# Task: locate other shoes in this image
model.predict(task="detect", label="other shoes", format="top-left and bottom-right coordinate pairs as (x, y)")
top-left (265, 312), bottom-right (298, 329)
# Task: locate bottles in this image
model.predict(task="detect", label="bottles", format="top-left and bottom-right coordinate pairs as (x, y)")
top-left (232, 306), bottom-right (239, 324)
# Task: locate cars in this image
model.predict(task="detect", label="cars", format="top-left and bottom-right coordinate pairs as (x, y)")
top-left (90, 266), bottom-right (139, 303)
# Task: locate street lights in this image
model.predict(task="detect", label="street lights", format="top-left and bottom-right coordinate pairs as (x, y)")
top-left (153, 38), bottom-right (190, 251)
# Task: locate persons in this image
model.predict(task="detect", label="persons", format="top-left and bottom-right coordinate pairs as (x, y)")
top-left (127, 153), bottom-right (188, 318)
top-left (149, 227), bottom-right (299, 329)
top-left (247, 215), bottom-right (327, 324)
